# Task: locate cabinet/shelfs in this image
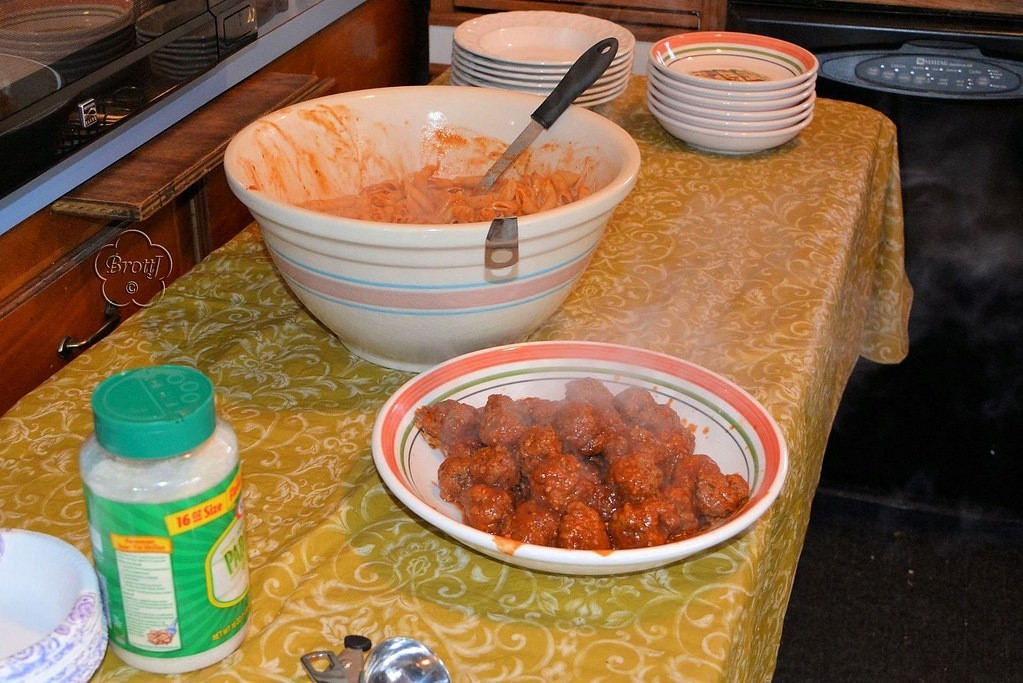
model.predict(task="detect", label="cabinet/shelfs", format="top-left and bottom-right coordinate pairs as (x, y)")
top-left (0, 191), bottom-right (201, 432)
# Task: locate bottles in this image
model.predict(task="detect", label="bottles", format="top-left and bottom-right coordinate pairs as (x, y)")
top-left (78, 365), bottom-right (251, 674)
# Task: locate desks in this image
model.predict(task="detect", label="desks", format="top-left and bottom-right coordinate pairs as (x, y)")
top-left (1, 66), bottom-right (913, 683)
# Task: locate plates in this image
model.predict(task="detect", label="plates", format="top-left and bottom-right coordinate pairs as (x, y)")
top-left (448, 10), bottom-right (635, 114)
top-left (648, 31), bottom-right (818, 156)
top-left (134, 1), bottom-right (253, 82)
top-left (370, 341), bottom-right (791, 576)
top-left (1, 5), bottom-right (133, 83)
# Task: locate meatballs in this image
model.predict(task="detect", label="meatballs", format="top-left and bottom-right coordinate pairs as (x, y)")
top-left (419, 378), bottom-right (750, 550)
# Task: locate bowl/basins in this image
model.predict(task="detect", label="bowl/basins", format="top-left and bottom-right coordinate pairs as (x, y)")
top-left (0, 528), bottom-right (108, 683)
top-left (223, 85), bottom-right (641, 374)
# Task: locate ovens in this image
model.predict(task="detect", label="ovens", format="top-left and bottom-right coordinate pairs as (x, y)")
top-left (724, 1), bottom-right (1023, 532)
